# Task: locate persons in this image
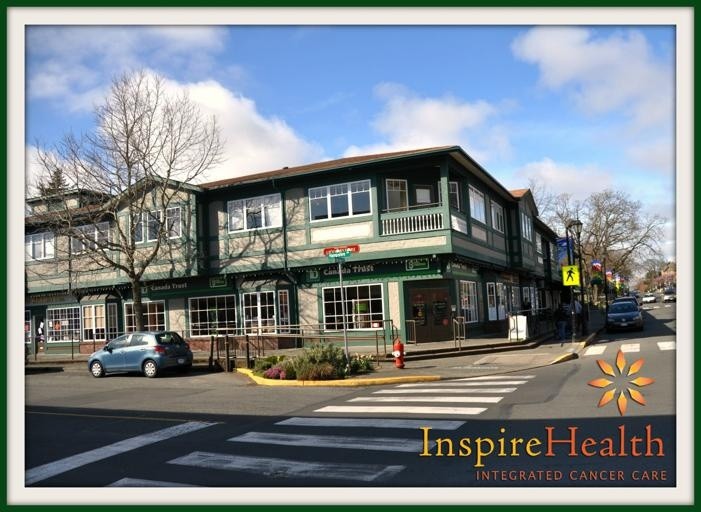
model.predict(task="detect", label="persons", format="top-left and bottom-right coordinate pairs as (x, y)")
top-left (555, 303), bottom-right (570, 343)
top-left (521, 296), bottom-right (532, 317)
top-left (568, 296), bottom-right (583, 335)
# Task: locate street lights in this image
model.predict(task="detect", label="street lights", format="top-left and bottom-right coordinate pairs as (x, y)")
top-left (572, 219), bottom-right (591, 337)
top-left (601, 247), bottom-right (611, 328)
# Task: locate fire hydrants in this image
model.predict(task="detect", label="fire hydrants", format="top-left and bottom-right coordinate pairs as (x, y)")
top-left (393, 340), bottom-right (407, 369)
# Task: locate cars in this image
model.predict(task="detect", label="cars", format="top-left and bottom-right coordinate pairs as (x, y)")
top-left (616, 296), bottom-right (639, 305)
top-left (607, 300), bottom-right (644, 333)
top-left (664, 291), bottom-right (677, 303)
top-left (643, 292), bottom-right (657, 304)
top-left (87, 330), bottom-right (195, 378)
